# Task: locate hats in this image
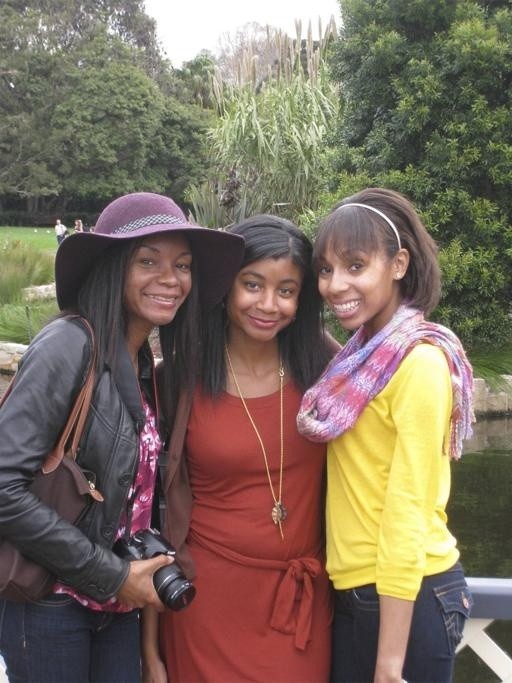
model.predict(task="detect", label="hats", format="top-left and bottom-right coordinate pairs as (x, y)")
top-left (55, 192), bottom-right (246, 329)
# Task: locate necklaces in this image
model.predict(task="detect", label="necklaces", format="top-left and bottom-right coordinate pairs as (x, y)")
top-left (222, 325), bottom-right (285, 540)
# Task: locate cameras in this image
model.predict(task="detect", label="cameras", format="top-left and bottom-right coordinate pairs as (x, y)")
top-left (112, 528), bottom-right (196, 611)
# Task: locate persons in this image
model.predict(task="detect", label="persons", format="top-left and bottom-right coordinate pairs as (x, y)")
top-left (55, 219), bottom-right (66, 243)
top-left (140, 214), bottom-right (333, 683)
top-left (74, 219), bottom-right (83, 234)
top-left (0, 192), bottom-right (246, 683)
top-left (311, 186), bottom-right (470, 683)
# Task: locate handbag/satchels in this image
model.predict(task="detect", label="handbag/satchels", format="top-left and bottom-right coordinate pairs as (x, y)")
top-left (1, 453), bottom-right (97, 603)
